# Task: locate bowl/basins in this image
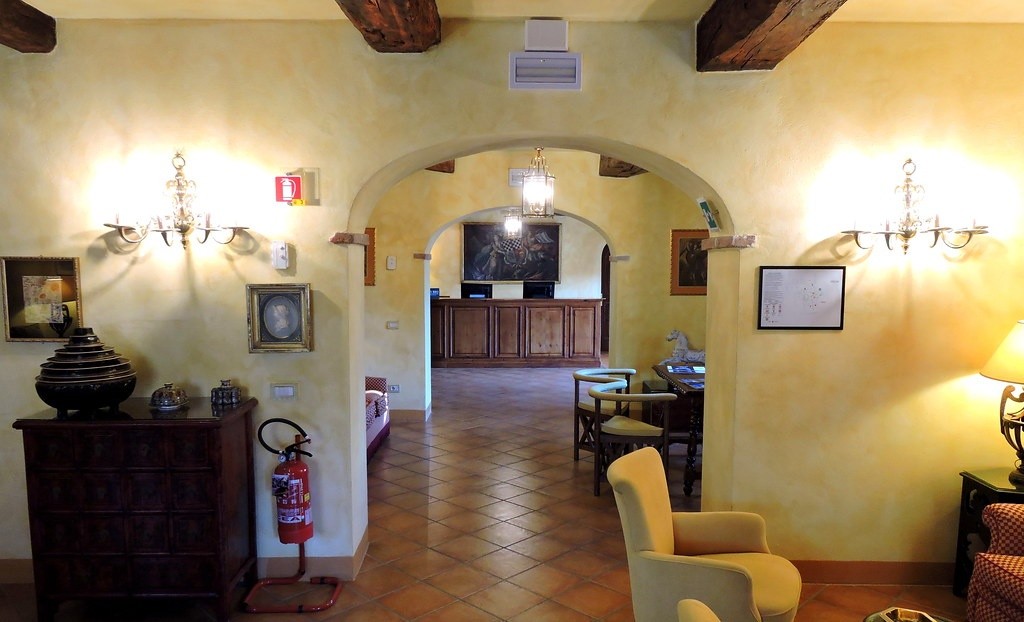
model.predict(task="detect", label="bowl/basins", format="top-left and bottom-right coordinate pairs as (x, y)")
top-left (878, 606), bottom-right (938, 622)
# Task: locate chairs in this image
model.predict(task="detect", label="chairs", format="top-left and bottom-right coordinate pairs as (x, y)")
top-left (574, 368), bottom-right (677, 497)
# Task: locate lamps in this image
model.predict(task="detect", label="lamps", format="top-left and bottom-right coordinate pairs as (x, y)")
top-left (980, 320), bottom-right (1024, 485)
top-left (103, 151), bottom-right (249, 250)
top-left (501, 147), bottom-right (556, 241)
top-left (840, 159), bottom-right (988, 255)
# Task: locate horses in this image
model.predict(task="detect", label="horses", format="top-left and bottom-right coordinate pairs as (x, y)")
top-left (657, 330), bottom-right (707, 366)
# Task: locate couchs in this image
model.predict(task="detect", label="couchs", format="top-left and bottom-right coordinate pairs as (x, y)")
top-left (607, 447), bottom-right (802, 622)
top-left (966, 503), bottom-right (1024, 621)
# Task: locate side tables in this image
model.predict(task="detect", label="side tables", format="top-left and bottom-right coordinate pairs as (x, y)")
top-left (953, 467), bottom-right (1024, 598)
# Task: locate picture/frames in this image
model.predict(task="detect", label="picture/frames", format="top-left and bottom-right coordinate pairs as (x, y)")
top-left (671, 230), bottom-right (709, 295)
top-left (246, 282), bottom-right (312, 353)
top-left (364, 228), bottom-right (375, 286)
top-left (757, 266), bottom-right (846, 330)
top-left (0, 256), bottom-right (83, 343)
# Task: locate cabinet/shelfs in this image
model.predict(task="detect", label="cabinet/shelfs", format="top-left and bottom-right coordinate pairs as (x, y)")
top-left (13, 397), bottom-right (258, 622)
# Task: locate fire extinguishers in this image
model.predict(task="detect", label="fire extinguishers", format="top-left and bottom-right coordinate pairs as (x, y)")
top-left (258, 418), bottom-right (314, 544)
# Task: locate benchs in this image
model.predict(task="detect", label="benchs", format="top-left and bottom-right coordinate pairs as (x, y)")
top-left (365, 377), bottom-right (390, 462)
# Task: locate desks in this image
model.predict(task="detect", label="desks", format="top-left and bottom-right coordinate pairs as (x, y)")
top-left (652, 362), bottom-right (705, 497)
top-left (861, 606), bottom-right (956, 622)
